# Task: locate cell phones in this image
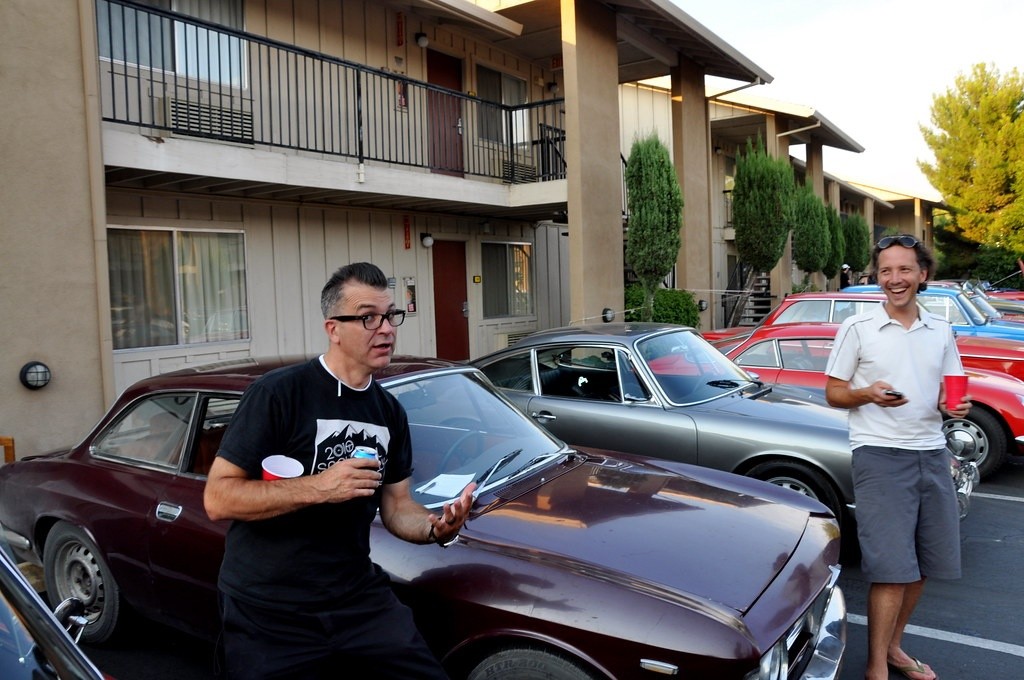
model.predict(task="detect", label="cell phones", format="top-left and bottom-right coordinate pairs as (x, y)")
top-left (885, 390), bottom-right (903, 399)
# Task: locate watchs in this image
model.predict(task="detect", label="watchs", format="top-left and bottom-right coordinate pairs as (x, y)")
top-left (427, 515), bottom-right (459, 550)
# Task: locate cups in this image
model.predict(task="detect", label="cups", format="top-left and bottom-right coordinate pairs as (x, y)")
top-left (261, 455), bottom-right (305, 481)
top-left (942, 372), bottom-right (969, 412)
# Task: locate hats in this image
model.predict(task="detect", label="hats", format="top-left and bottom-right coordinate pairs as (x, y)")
top-left (842, 264), bottom-right (850, 270)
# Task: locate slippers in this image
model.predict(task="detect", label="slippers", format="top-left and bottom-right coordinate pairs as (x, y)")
top-left (886, 656), bottom-right (940, 680)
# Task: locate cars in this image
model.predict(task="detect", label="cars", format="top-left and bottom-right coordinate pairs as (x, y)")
top-left (925, 276), bottom-right (1024, 323)
top-left (468, 322), bottom-right (972, 562)
top-left (0, 539), bottom-right (105, 680)
top-left (843, 282), bottom-right (1022, 340)
top-left (0, 343), bottom-right (852, 680)
top-left (706, 325), bottom-right (1022, 482)
top-left (703, 289), bottom-right (1023, 382)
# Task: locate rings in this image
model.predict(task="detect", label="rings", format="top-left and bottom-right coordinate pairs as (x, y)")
top-left (444, 516), bottom-right (457, 526)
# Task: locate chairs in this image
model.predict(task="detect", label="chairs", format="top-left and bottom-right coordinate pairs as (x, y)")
top-left (610, 369), bottom-right (650, 401)
top-left (411, 423), bottom-right (460, 482)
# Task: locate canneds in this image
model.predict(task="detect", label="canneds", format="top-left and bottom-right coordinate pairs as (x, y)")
top-left (351, 447), bottom-right (378, 482)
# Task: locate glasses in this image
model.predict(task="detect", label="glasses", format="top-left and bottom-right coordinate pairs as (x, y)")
top-left (877, 236), bottom-right (917, 249)
top-left (329, 309), bottom-right (406, 330)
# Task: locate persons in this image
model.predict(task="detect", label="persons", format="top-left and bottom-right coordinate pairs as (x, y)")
top-left (826, 235), bottom-right (973, 680)
top-left (203, 262), bottom-right (477, 680)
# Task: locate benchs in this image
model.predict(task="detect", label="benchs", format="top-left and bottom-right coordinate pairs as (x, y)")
top-left (120, 412), bottom-right (229, 476)
top-left (493, 368), bottom-right (601, 399)
